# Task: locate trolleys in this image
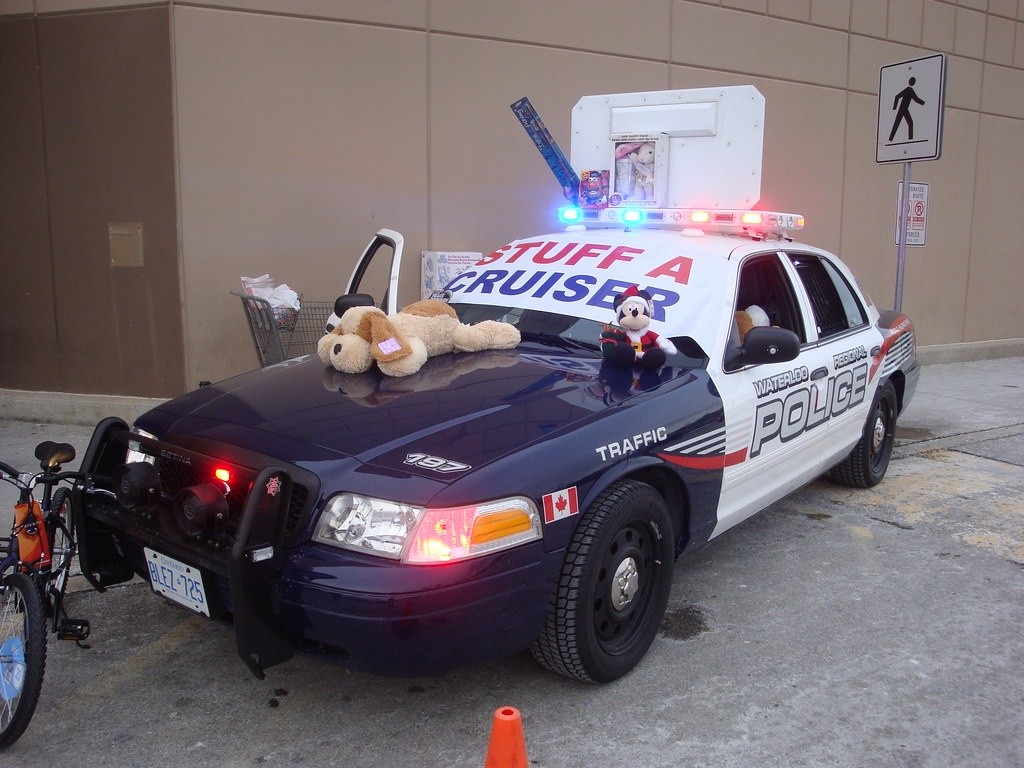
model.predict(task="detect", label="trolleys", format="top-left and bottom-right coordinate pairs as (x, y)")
top-left (226, 289), bottom-right (403, 367)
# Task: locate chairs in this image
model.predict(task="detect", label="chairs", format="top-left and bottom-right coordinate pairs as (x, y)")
top-left (737, 300), bottom-right (773, 326)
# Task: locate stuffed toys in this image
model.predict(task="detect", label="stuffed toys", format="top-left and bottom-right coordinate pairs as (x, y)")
top-left (318, 300), bottom-right (521, 376)
top-left (735, 310), bottom-right (754, 343)
top-left (598, 367), bottom-right (661, 406)
top-left (628, 143), bottom-right (654, 199)
top-left (600, 285), bottom-right (676, 367)
top-left (322, 348), bottom-right (520, 407)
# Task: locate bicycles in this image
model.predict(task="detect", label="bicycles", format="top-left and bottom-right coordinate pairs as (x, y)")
top-left (1, 442), bottom-right (119, 753)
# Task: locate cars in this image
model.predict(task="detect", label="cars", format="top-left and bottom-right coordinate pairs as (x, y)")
top-left (69, 201), bottom-right (920, 686)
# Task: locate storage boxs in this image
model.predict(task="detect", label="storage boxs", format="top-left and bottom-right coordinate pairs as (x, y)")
top-left (578, 169), bottom-right (610, 209)
top-left (609, 132), bottom-right (670, 208)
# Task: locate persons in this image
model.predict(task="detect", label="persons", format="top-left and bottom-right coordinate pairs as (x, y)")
top-left (579, 169), bottom-right (609, 207)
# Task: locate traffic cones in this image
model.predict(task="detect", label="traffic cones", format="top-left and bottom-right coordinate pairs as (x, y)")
top-left (483, 706), bottom-right (529, 767)
